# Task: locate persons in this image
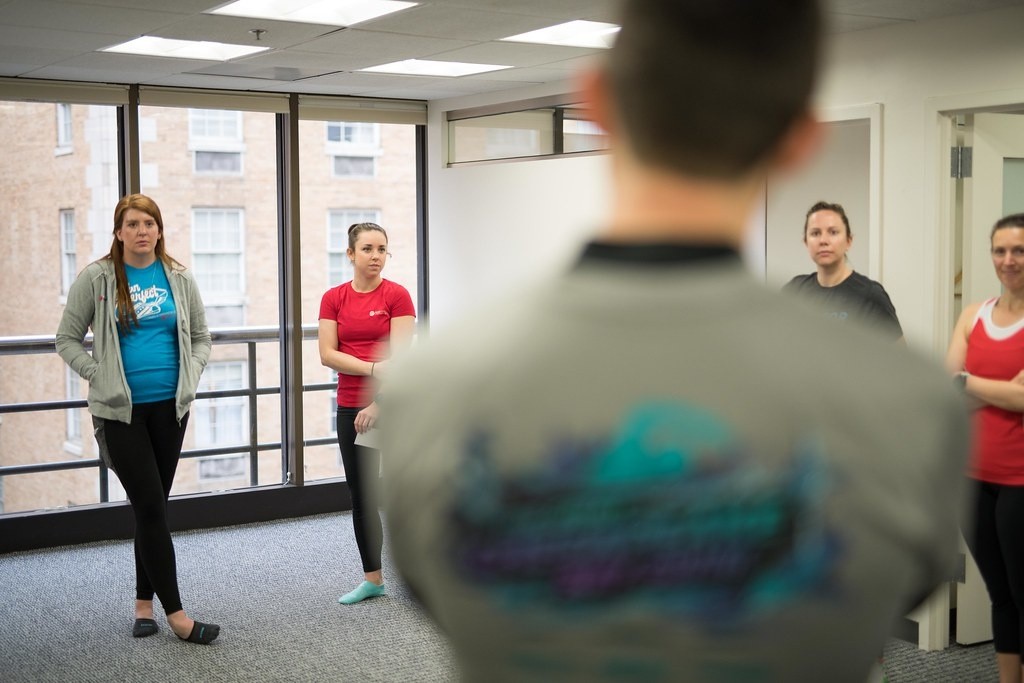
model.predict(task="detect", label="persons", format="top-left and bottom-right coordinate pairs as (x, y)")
top-left (945, 213), bottom-right (1023, 682)
top-left (374, 0), bottom-right (973, 683)
top-left (56, 194), bottom-right (219, 644)
top-left (319, 222), bottom-right (416, 605)
top-left (778, 200), bottom-right (907, 343)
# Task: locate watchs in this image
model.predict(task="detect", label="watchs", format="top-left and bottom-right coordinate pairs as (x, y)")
top-left (954, 373), bottom-right (967, 389)
